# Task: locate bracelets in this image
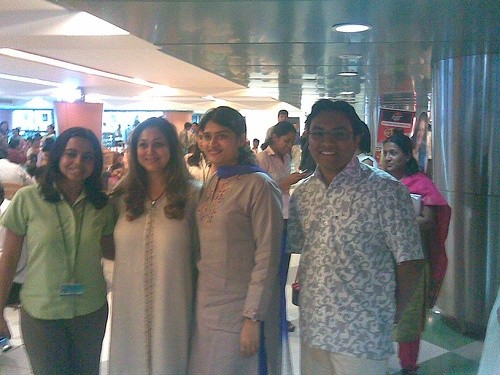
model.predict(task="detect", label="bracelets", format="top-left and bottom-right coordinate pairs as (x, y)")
top-left (247, 317), bottom-right (260, 322)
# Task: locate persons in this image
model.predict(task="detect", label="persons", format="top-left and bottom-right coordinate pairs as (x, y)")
top-left (0, 98), bottom-right (440, 375)
top-left (410, 112), bottom-right (429, 175)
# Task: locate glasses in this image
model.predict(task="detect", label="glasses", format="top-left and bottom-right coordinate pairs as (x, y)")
top-left (306, 129), bottom-right (357, 141)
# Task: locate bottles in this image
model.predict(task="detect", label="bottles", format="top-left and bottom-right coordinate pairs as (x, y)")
top-left (0, 334), bottom-right (12, 352)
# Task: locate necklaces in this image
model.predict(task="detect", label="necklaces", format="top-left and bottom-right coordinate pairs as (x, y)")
top-left (146, 186), bottom-right (168, 207)
